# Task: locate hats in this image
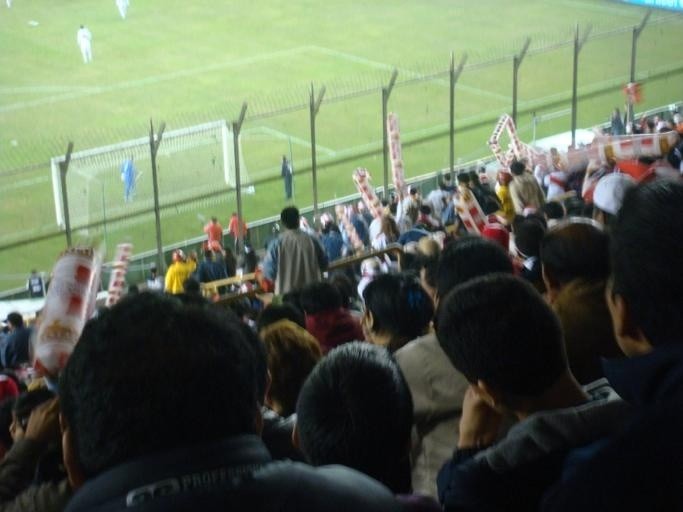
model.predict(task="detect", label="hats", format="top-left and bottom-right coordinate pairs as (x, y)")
top-left (482, 224), bottom-right (508, 251)
top-left (593, 173), bottom-right (635, 214)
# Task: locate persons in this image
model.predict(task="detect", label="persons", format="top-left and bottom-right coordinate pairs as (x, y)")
top-left (116, 0), bottom-right (129, 20)
top-left (77, 24), bottom-right (94, 64)
top-left (0, 100), bottom-right (682, 511)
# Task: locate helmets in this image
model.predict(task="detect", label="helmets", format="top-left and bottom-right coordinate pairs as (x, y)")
top-left (172, 249), bottom-right (186, 262)
